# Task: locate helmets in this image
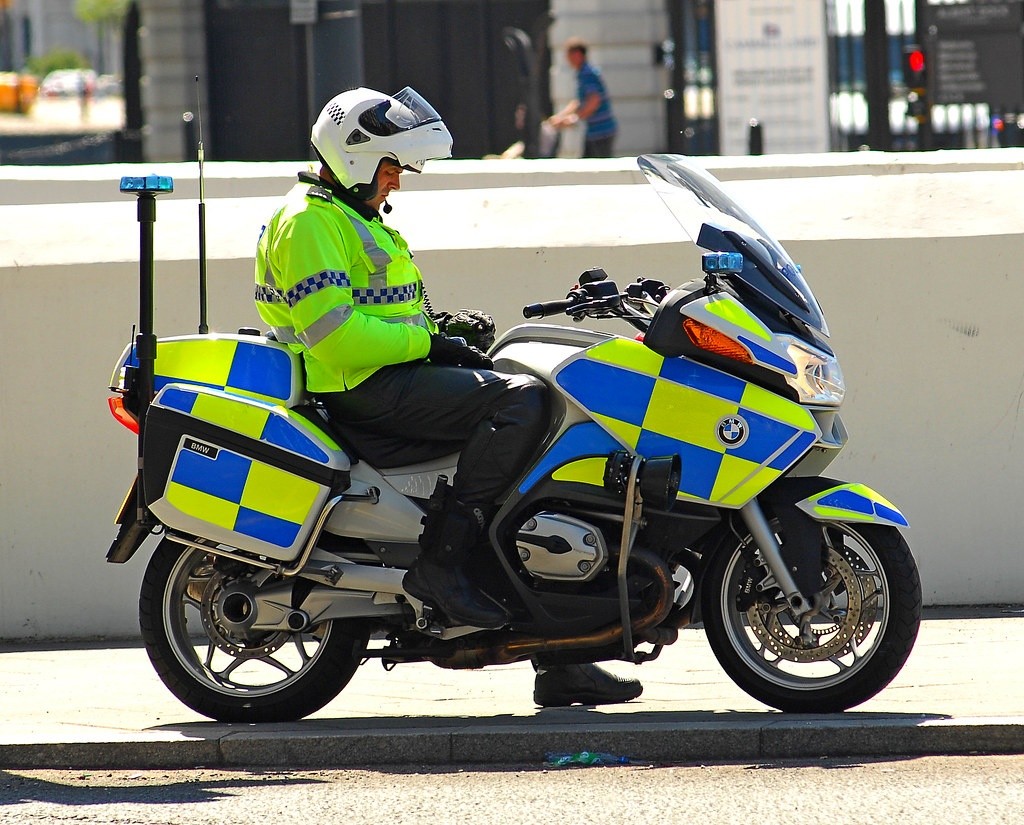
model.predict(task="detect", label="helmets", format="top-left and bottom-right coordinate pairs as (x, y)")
top-left (311, 85), bottom-right (453, 201)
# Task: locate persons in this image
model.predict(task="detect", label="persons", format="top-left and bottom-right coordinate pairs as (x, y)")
top-left (255, 87), bottom-right (644, 707)
top-left (547, 40), bottom-right (618, 158)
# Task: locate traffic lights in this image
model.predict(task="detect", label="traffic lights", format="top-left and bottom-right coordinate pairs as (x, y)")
top-left (902, 45), bottom-right (927, 121)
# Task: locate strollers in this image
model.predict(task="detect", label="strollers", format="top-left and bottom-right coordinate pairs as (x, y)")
top-left (499, 119), bottom-right (563, 159)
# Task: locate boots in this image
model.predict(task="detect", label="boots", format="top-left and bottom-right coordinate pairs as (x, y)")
top-left (534, 663), bottom-right (643, 708)
top-left (402, 500), bottom-right (507, 630)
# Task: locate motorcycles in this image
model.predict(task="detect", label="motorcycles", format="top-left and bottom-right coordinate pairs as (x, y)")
top-left (105, 143), bottom-right (924, 725)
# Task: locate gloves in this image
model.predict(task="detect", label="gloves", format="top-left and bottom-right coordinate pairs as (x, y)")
top-left (444, 309), bottom-right (496, 354)
top-left (430, 332), bottom-right (494, 370)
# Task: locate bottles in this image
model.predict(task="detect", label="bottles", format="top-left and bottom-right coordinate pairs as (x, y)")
top-left (550, 752), bottom-right (628, 764)
